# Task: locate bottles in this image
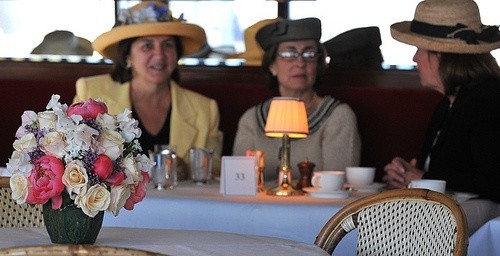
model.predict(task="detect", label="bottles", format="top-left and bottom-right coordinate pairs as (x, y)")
top-left (278, 164), bottom-right (292, 186)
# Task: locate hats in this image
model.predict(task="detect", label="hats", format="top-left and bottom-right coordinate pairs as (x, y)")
top-left (390, 0), bottom-right (500, 54)
top-left (256, 18), bottom-right (321, 51)
top-left (227, 19), bottom-right (288, 59)
top-left (92, 1), bottom-right (206, 61)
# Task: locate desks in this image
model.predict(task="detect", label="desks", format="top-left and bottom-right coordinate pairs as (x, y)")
top-left (0, 224), bottom-right (329, 256)
top-left (0, 166), bottom-right (385, 256)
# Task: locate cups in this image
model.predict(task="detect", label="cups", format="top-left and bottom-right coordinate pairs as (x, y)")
top-left (346, 167), bottom-right (376, 185)
top-left (189, 146), bottom-right (214, 185)
top-left (245, 149), bottom-right (265, 192)
top-left (408, 180), bottom-right (447, 194)
top-left (311, 171), bottom-right (345, 191)
top-left (152, 143), bottom-right (178, 190)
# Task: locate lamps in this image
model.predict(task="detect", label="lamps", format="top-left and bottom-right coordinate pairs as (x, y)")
top-left (265, 97), bottom-right (309, 198)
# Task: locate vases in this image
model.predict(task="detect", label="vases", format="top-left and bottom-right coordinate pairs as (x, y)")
top-left (40, 191), bottom-right (105, 244)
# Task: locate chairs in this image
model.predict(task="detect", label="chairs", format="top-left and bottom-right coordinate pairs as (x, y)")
top-left (314, 187), bottom-right (470, 256)
top-left (0, 176), bottom-right (45, 228)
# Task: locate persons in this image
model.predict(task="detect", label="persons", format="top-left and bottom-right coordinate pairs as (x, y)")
top-left (73, 0), bottom-right (223, 181)
top-left (382, 0), bottom-right (500, 204)
top-left (232, 18), bottom-right (361, 187)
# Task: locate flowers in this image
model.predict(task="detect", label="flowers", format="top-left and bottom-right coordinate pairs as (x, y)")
top-left (3, 92), bottom-right (157, 220)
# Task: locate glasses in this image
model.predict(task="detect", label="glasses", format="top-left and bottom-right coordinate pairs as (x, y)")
top-left (276, 48), bottom-right (320, 62)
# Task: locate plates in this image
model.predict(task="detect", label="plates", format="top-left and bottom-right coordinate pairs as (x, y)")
top-left (346, 184), bottom-right (387, 193)
top-left (302, 186), bottom-right (353, 196)
